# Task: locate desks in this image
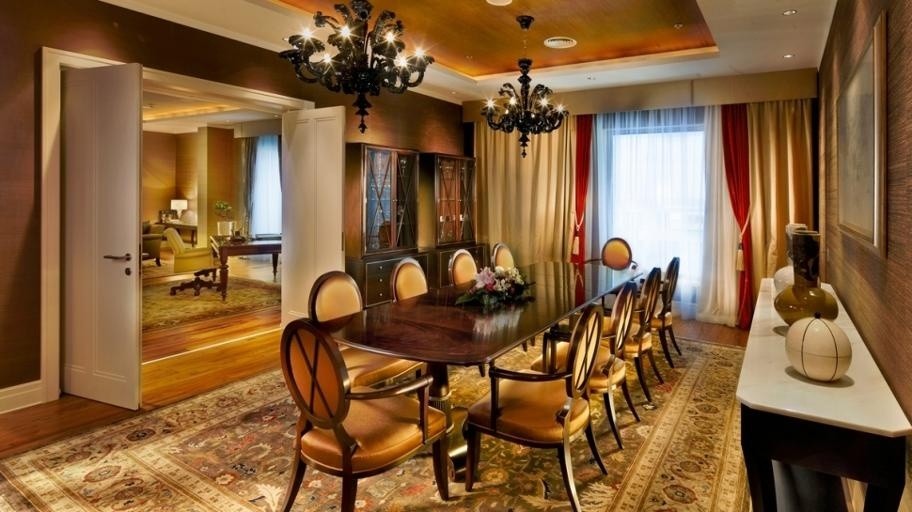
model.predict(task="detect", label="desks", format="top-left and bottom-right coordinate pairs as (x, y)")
top-left (156, 222), bottom-right (197, 248)
top-left (210, 234), bottom-right (281, 300)
top-left (733, 277), bottom-right (912, 512)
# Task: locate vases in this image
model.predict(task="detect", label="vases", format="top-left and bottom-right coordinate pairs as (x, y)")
top-left (774, 223), bottom-right (821, 292)
top-left (774, 231), bottom-right (839, 326)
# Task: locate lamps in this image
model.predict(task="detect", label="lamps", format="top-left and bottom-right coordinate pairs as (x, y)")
top-left (278, 0), bottom-right (434, 134)
top-left (171, 200), bottom-right (188, 219)
top-left (545, 37), bottom-right (577, 50)
top-left (486, 0), bottom-right (511, 6)
top-left (480, 16), bottom-right (570, 158)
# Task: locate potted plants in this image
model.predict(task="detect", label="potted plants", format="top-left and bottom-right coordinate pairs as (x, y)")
top-left (215, 200), bottom-right (235, 235)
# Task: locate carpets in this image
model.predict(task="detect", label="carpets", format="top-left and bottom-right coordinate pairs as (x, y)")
top-left (143, 259), bottom-right (220, 280)
top-left (143, 274), bottom-right (281, 335)
top-left (1, 329), bottom-right (754, 512)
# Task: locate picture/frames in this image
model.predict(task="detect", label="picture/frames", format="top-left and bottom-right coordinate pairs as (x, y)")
top-left (837, 13), bottom-right (887, 264)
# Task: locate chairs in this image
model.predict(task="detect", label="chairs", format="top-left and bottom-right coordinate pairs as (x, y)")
top-left (142, 224), bottom-right (163, 266)
top-left (164, 229), bottom-right (220, 295)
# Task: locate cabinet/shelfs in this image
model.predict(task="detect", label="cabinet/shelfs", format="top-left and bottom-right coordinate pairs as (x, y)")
top-left (418, 153), bottom-right (475, 249)
top-left (345, 145), bottom-right (417, 257)
